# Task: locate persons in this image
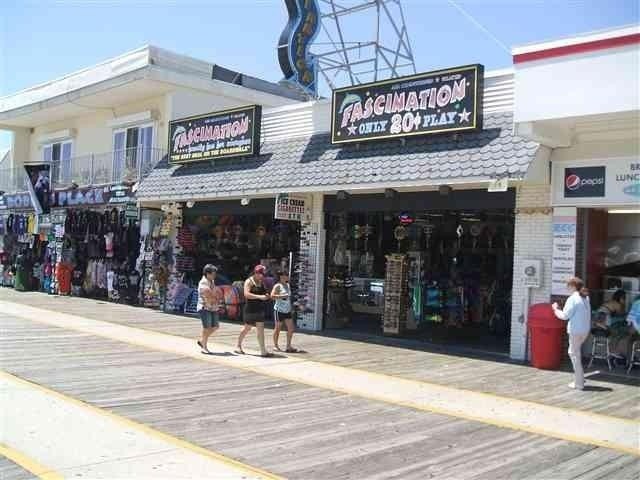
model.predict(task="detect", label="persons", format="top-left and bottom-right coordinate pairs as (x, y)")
top-left (196, 263), bottom-right (222, 355)
top-left (234, 265), bottom-right (276, 357)
top-left (591, 289), bottom-right (626, 371)
top-left (14, 249), bottom-right (28, 292)
top-left (623, 293), bottom-right (640, 369)
top-left (552, 276), bottom-right (591, 390)
top-left (270, 270), bottom-right (299, 353)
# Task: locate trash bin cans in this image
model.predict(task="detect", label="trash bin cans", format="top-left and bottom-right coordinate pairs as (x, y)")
top-left (527, 303), bottom-right (564, 370)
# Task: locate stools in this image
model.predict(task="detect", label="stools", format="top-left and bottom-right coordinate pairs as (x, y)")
top-left (624, 333), bottom-right (640, 377)
top-left (586, 327), bottom-right (620, 372)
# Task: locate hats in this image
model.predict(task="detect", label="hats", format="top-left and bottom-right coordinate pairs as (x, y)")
top-left (254, 265), bottom-right (266, 273)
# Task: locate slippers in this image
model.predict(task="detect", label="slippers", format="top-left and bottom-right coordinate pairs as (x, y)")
top-left (235, 350), bottom-right (245, 355)
top-left (261, 353), bottom-right (274, 358)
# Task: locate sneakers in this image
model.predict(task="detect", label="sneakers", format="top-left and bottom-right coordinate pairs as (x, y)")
top-left (569, 382), bottom-right (585, 390)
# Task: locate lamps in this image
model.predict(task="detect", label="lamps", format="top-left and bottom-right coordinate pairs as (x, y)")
top-left (186, 200), bottom-right (195, 209)
top-left (239, 198), bottom-right (250, 208)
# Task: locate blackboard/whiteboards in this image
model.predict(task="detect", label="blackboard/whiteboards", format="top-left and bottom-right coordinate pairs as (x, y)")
top-left (184, 288), bottom-right (200, 315)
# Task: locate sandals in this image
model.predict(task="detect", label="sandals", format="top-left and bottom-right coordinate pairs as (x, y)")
top-left (274, 348), bottom-right (299, 352)
top-left (198, 341), bottom-right (211, 354)
